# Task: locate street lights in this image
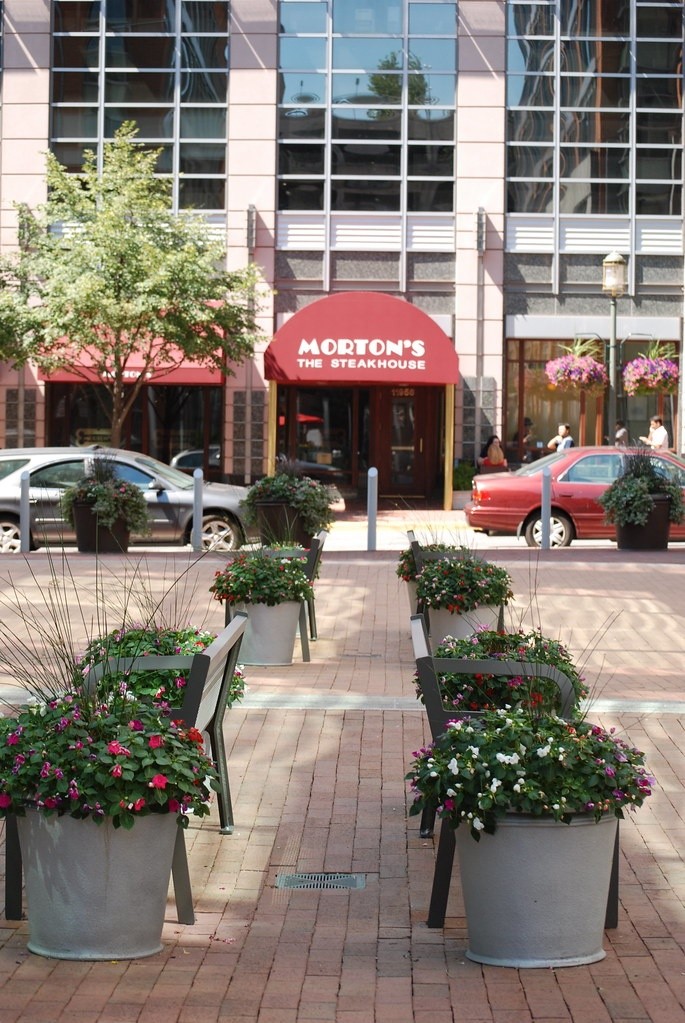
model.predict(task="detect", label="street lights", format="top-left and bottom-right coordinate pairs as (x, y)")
top-left (602, 249), bottom-right (628, 443)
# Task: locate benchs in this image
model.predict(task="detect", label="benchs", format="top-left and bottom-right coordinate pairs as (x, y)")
top-left (225, 529), bottom-right (324, 663)
top-left (81, 614), bottom-right (249, 926)
top-left (409, 619), bottom-right (622, 928)
top-left (406, 530), bottom-right (449, 576)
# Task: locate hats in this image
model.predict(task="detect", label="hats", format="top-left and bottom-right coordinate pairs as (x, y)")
top-left (524, 417), bottom-right (533, 425)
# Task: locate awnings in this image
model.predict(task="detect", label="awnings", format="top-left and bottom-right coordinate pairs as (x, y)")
top-left (264, 292), bottom-right (459, 388)
top-left (37, 298), bottom-right (227, 387)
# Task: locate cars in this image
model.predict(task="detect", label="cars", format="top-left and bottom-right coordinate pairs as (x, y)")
top-left (462, 444), bottom-right (685, 550)
top-left (170, 444), bottom-right (345, 491)
top-left (0, 444), bottom-right (263, 554)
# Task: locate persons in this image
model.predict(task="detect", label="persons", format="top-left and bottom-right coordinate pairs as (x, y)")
top-left (476, 415), bottom-right (668, 473)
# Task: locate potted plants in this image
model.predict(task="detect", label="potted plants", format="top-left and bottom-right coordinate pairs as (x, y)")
top-left (601, 438), bottom-right (685, 551)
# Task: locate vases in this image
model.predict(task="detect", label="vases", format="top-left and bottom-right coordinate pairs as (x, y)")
top-left (73, 505), bottom-right (128, 553)
top-left (228, 598), bottom-right (300, 667)
top-left (405, 580), bottom-right (418, 616)
top-left (253, 499), bottom-right (302, 544)
top-left (454, 815), bottom-right (619, 970)
top-left (16, 806), bottom-right (181, 961)
top-left (427, 602), bottom-right (501, 650)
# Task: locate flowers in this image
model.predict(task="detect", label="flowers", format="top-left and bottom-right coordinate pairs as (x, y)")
top-left (412, 630), bottom-right (588, 717)
top-left (80, 623), bottom-right (244, 713)
top-left (208, 553), bottom-right (315, 607)
top-left (621, 358), bottom-right (679, 395)
top-left (59, 452), bottom-right (149, 535)
top-left (416, 554), bottom-right (514, 616)
top-left (402, 711), bottom-right (658, 836)
top-left (238, 473), bottom-right (340, 536)
top-left (396, 544), bottom-right (456, 581)
top-left (545, 353), bottom-right (609, 394)
top-left (0, 677), bottom-right (223, 830)
